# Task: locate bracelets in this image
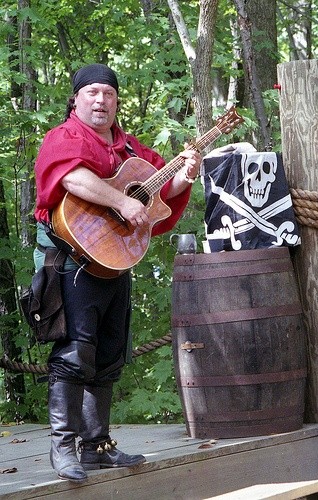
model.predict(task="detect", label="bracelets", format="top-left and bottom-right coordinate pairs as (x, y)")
top-left (182, 166), bottom-right (200, 184)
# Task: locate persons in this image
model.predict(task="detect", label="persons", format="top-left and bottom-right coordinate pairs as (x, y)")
top-left (33, 64), bottom-right (202, 482)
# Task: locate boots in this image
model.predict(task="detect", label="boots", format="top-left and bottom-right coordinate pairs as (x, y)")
top-left (76, 357), bottom-right (146, 470)
top-left (46, 340), bottom-right (96, 483)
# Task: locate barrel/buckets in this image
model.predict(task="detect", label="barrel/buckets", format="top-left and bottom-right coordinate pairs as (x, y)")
top-left (171, 247), bottom-right (307, 437)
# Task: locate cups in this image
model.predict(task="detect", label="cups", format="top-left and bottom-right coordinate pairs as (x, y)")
top-left (170, 233), bottom-right (197, 255)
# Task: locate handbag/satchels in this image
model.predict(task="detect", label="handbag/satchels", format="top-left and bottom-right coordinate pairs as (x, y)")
top-left (17, 247), bottom-right (68, 345)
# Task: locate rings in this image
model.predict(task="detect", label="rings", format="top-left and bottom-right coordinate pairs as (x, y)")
top-left (137, 220), bottom-right (143, 223)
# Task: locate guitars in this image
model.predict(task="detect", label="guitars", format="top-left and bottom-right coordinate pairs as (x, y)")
top-left (49, 102), bottom-right (246, 281)
